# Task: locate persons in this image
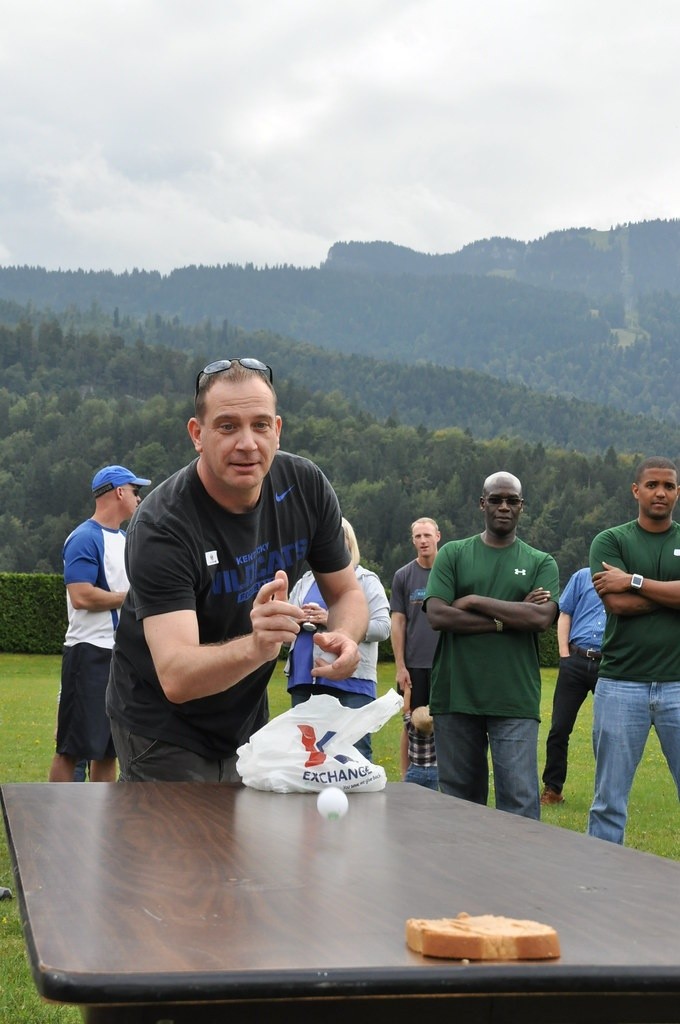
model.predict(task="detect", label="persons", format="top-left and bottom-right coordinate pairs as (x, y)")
top-left (587, 456), bottom-right (680, 845)
top-left (50, 465), bottom-right (151, 782)
top-left (540, 568), bottom-right (607, 806)
top-left (105, 357), bottom-right (368, 783)
top-left (287, 517), bottom-right (392, 764)
top-left (389, 517), bottom-right (441, 781)
top-left (402, 682), bottom-right (439, 792)
top-left (420, 471), bottom-right (560, 820)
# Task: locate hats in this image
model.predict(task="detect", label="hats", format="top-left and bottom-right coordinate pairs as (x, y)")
top-left (92, 464), bottom-right (152, 500)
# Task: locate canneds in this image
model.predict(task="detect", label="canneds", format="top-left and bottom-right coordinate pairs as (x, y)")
top-left (303, 602), bottom-right (320, 631)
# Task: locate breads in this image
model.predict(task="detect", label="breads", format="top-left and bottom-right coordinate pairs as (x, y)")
top-left (405, 913), bottom-right (561, 958)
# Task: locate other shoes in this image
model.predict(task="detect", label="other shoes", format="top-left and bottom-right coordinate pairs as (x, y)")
top-left (540, 784), bottom-right (566, 808)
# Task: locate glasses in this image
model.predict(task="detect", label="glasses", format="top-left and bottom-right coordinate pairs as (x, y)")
top-left (110, 487), bottom-right (139, 498)
top-left (195, 358), bottom-right (273, 406)
top-left (481, 495), bottom-right (523, 506)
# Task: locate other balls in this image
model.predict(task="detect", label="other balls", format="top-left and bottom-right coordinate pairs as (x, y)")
top-left (320, 650), bottom-right (339, 664)
top-left (317, 787), bottom-right (348, 821)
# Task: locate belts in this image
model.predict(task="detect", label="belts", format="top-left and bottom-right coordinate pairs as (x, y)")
top-left (569, 643), bottom-right (602, 661)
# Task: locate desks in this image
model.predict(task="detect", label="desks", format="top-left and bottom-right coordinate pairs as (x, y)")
top-left (0, 773), bottom-right (680, 1024)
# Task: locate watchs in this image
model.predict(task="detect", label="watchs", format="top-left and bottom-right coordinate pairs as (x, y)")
top-left (493, 618), bottom-right (503, 631)
top-left (630, 573), bottom-right (643, 589)
